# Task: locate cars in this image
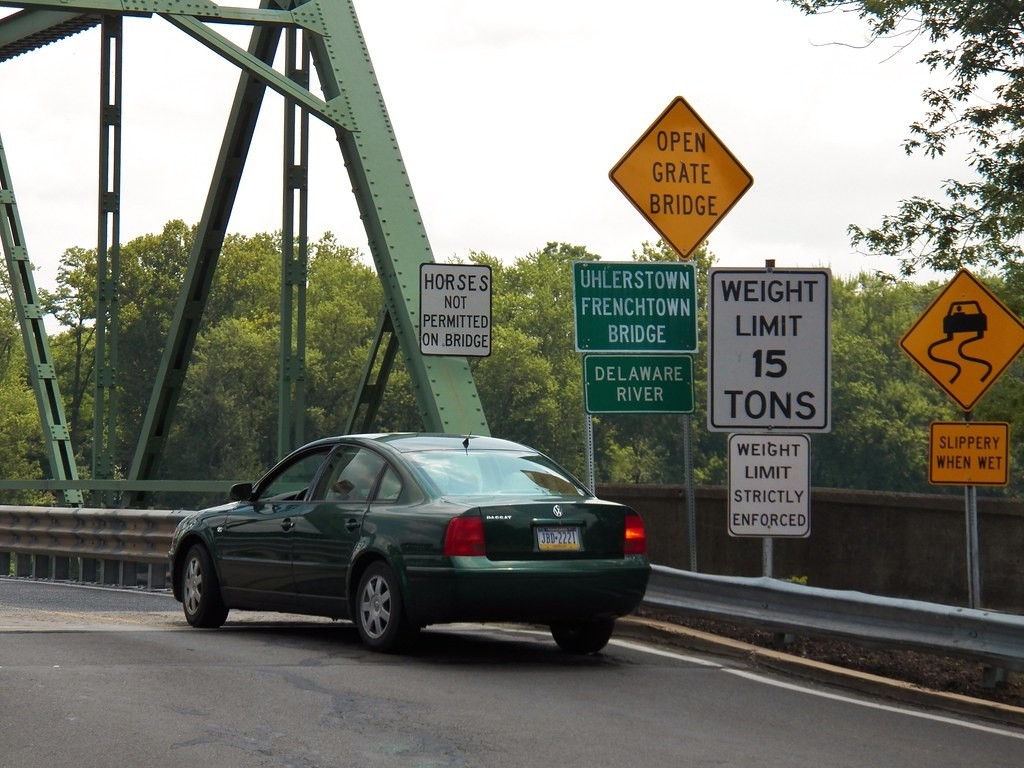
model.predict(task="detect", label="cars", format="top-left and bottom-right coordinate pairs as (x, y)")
top-left (165, 433), bottom-right (652, 657)
